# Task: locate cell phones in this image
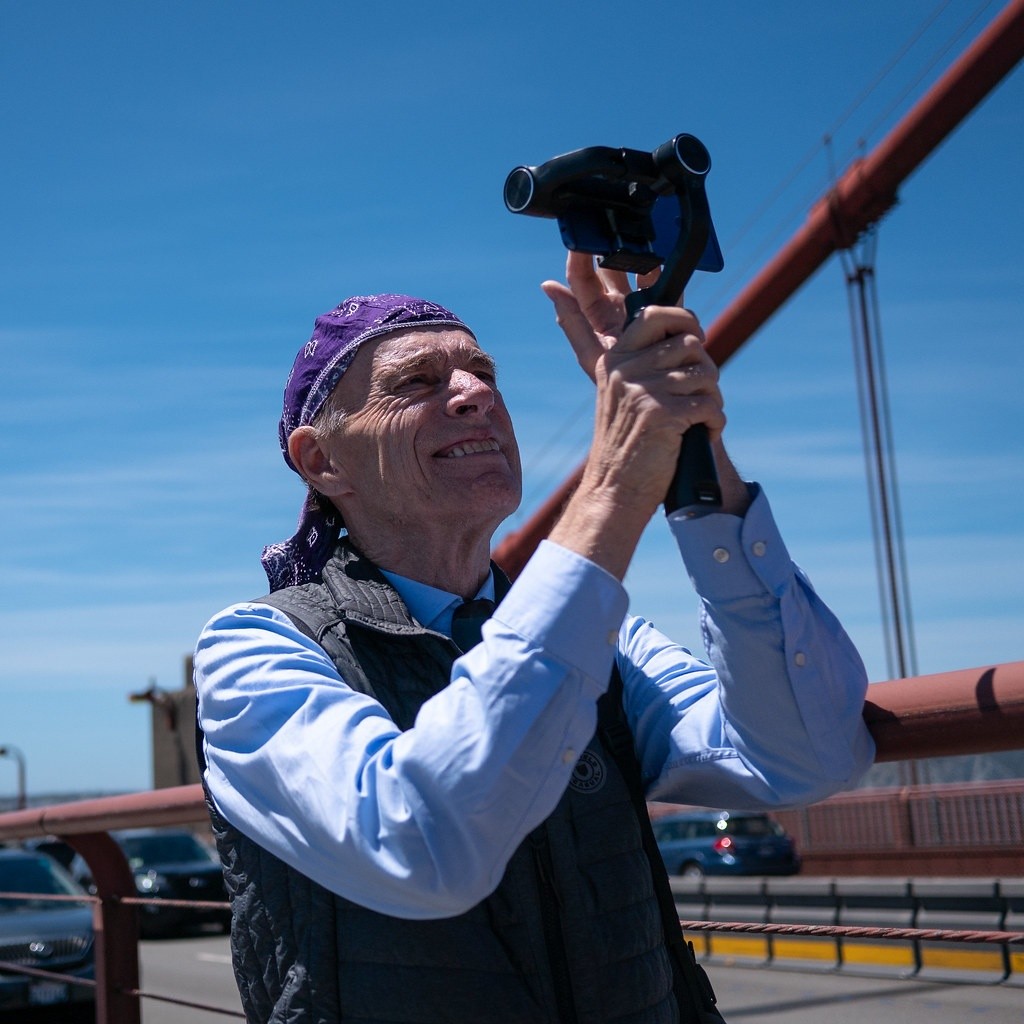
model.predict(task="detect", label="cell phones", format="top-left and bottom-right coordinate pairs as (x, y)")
top-left (557, 191), bottom-right (724, 273)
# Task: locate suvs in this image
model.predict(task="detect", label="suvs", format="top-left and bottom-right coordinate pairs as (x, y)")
top-left (648, 810), bottom-right (800, 878)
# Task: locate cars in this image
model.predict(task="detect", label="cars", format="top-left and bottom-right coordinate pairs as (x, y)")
top-left (65, 829), bottom-right (234, 941)
top-left (1, 847), bottom-right (99, 1024)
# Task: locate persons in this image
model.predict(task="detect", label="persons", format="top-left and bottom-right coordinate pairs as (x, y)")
top-left (193, 250), bottom-right (878, 1024)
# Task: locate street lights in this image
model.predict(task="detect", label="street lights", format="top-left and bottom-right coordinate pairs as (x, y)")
top-left (0, 743), bottom-right (27, 810)
